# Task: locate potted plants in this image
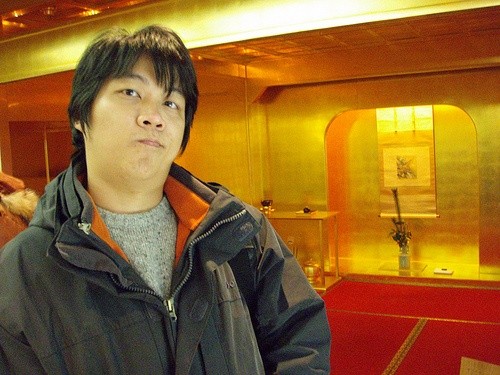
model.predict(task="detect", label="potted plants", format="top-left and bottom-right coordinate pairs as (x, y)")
top-left (386, 186), bottom-right (414, 273)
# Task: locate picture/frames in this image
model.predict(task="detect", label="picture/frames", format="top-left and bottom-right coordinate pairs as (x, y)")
top-left (372, 105), bottom-right (441, 221)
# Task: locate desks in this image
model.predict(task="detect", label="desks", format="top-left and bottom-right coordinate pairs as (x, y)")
top-left (257, 209), bottom-right (343, 291)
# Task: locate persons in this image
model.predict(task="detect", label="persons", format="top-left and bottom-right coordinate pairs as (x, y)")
top-left (0, 24), bottom-right (331, 375)
top-left (0, 171), bottom-right (38, 248)
